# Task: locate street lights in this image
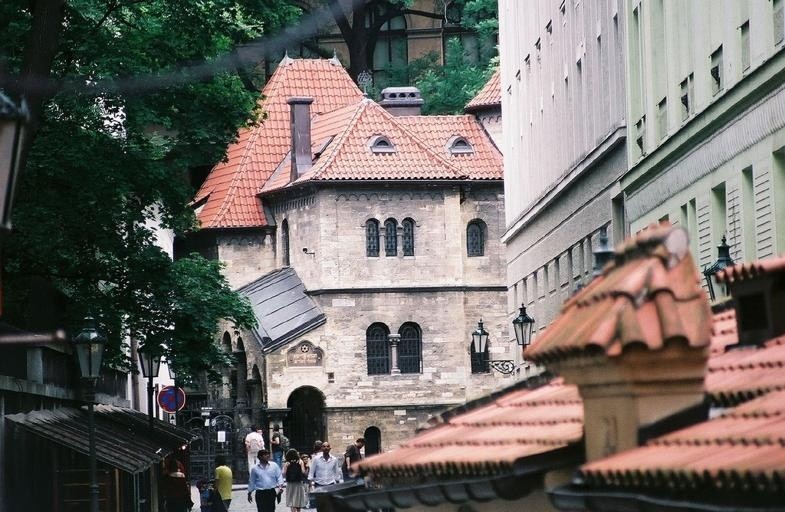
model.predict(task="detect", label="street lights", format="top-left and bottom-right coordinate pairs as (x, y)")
top-left (73, 308), bottom-right (110, 511)
top-left (165, 352), bottom-right (184, 426)
top-left (134, 328), bottom-right (160, 510)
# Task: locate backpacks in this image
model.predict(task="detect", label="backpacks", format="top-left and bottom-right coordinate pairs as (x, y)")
top-left (285, 459), bottom-right (304, 482)
top-left (275, 431), bottom-right (290, 452)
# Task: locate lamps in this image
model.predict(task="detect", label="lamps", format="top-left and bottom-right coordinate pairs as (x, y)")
top-left (511, 300), bottom-right (536, 353)
top-left (472, 315), bottom-right (517, 376)
top-left (701, 235), bottom-right (737, 302)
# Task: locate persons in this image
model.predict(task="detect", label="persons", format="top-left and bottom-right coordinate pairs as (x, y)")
top-left (245, 424), bottom-right (264, 477)
top-left (300, 454), bottom-right (312, 509)
top-left (269, 423), bottom-right (283, 469)
top-left (343, 438), bottom-right (366, 480)
top-left (310, 439), bottom-right (323, 508)
top-left (305, 442), bottom-right (341, 487)
top-left (212, 453), bottom-right (234, 512)
top-left (247, 449), bottom-right (285, 512)
top-left (282, 448), bottom-right (310, 512)
